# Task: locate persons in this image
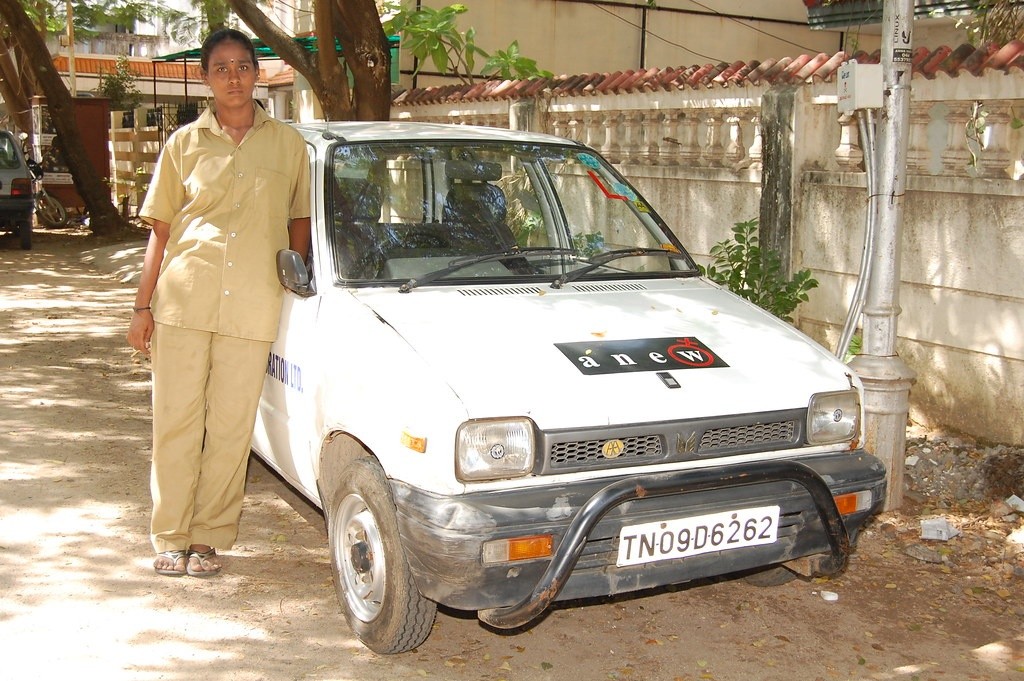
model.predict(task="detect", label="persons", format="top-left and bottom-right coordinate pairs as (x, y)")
top-left (127, 29), bottom-right (310, 577)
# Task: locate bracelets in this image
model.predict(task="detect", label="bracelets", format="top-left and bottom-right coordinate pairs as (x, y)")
top-left (133, 307), bottom-right (151, 311)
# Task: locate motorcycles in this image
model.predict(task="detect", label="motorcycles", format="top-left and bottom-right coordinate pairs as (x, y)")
top-left (12, 132), bottom-right (69, 229)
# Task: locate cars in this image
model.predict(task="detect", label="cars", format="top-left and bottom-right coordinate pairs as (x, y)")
top-left (0, 131), bottom-right (33, 250)
top-left (249, 120), bottom-right (887, 656)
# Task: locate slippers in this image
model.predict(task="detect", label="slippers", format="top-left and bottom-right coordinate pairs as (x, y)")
top-left (155, 550), bottom-right (187, 575)
top-left (186, 547), bottom-right (221, 576)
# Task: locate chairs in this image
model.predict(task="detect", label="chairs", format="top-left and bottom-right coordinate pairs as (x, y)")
top-left (332, 176), bottom-right (389, 279)
top-left (440, 181), bottom-right (516, 252)
